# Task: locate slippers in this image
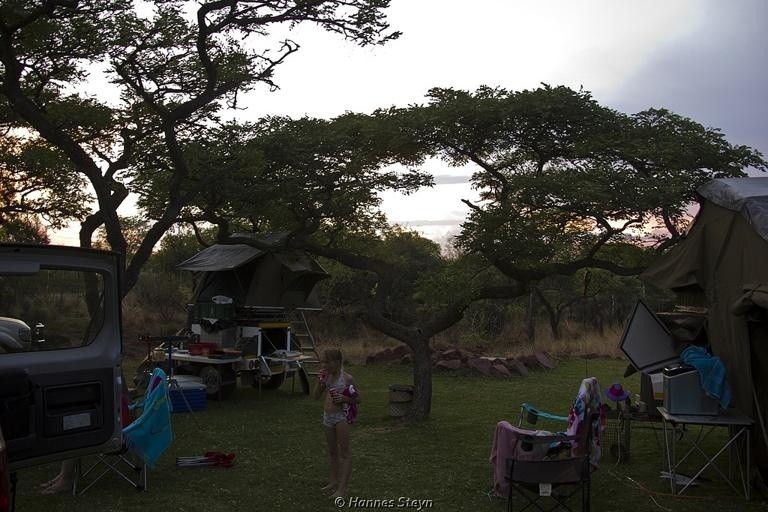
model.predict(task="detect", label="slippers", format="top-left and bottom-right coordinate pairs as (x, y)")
top-left (40, 481), bottom-right (66, 496)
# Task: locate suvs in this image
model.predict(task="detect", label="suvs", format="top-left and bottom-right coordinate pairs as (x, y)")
top-left (133, 314), bottom-right (305, 401)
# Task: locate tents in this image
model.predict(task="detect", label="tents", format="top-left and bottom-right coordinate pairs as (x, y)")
top-left (637, 176), bottom-right (768, 503)
top-left (174, 235), bottom-right (330, 308)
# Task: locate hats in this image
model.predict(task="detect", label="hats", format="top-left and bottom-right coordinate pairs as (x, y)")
top-left (604, 384), bottom-right (629, 401)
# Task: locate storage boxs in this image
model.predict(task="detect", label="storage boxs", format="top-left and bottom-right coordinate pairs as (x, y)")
top-left (189, 343), bottom-right (216, 355)
top-left (168, 382), bottom-right (207, 413)
top-left (167, 375), bottom-right (203, 383)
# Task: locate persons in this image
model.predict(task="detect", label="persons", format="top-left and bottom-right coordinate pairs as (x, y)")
top-left (312, 345), bottom-right (357, 498)
top-left (39, 393), bottom-right (130, 497)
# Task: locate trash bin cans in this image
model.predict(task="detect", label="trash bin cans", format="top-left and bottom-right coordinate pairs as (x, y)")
top-left (388, 384), bottom-right (414, 416)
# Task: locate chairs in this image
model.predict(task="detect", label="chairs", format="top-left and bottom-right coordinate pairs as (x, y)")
top-left (72, 367), bottom-right (173, 497)
top-left (504, 403), bottom-right (595, 512)
top-left (494, 377), bottom-right (605, 498)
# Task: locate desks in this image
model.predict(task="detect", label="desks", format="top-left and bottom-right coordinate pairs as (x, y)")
top-left (656, 407), bottom-right (755, 501)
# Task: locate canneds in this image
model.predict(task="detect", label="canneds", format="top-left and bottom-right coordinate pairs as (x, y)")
top-left (319, 369), bottom-right (328, 384)
top-left (329, 388), bottom-right (341, 404)
